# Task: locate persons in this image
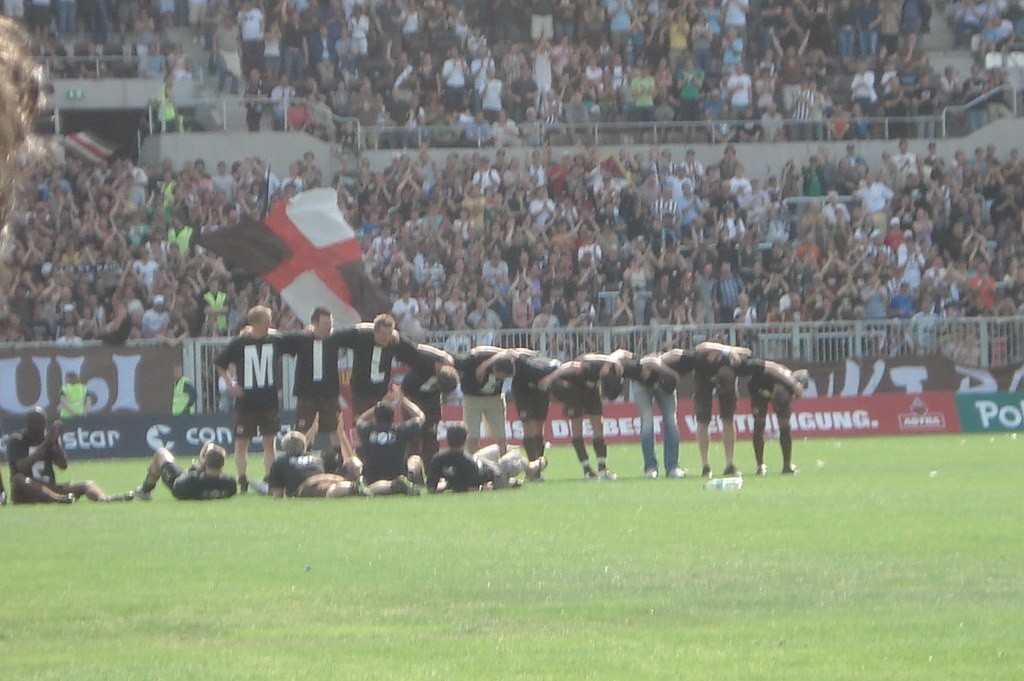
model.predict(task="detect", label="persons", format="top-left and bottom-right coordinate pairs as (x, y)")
top-left (0, 1), bottom-right (1023, 367)
top-left (0, 306), bottom-right (809, 506)
top-left (55, 371), bottom-right (91, 420)
top-left (169, 364), bottom-right (196, 415)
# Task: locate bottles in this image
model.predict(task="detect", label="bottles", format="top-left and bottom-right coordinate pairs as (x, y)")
top-left (703, 477), bottom-right (743, 491)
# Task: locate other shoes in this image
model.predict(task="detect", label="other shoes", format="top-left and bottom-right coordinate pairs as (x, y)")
top-left (783, 466), bottom-right (794, 474)
top-left (701, 464), bottom-right (713, 479)
top-left (598, 468), bottom-right (617, 480)
top-left (62, 493), bottom-right (76, 505)
top-left (646, 465), bottom-right (658, 480)
top-left (525, 456), bottom-right (548, 479)
top-left (110, 493), bottom-right (125, 503)
top-left (757, 464), bottom-right (767, 476)
top-left (129, 487), bottom-right (152, 502)
top-left (667, 467), bottom-right (685, 477)
top-left (584, 468), bottom-right (598, 479)
top-left (724, 465), bottom-right (743, 477)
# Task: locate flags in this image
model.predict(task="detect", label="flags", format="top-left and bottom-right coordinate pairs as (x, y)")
top-left (61, 132), bottom-right (120, 164)
top-left (196, 186), bottom-right (381, 335)
top-left (599, 159), bottom-right (629, 182)
